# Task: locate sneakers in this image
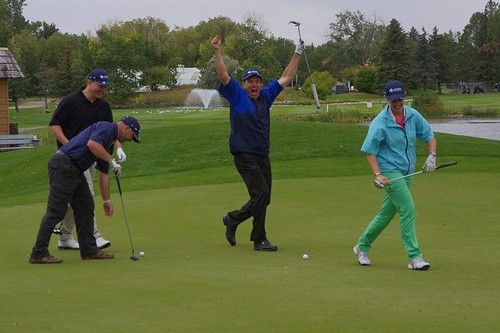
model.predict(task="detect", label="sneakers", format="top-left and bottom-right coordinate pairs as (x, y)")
top-left (353, 245), bottom-right (371, 265)
top-left (57, 238), bottom-right (80, 251)
top-left (406, 257), bottom-right (430, 271)
top-left (96, 237), bottom-right (111, 249)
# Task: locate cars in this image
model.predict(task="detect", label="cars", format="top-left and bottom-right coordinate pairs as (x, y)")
top-left (462, 87), bottom-right (470, 94)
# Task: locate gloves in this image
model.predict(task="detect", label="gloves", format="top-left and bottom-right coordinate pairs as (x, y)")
top-left (108, 158), bottom-right (122, 178)
top-left (423, 152), bottom-right (436, 172)
top-left (116, 147), bottom-right (127, 162)
top-left (295, 38), bottom-right (306, 56)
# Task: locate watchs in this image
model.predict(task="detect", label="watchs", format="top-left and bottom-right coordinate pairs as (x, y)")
top-left (430, 152), bottom-right (437, 156)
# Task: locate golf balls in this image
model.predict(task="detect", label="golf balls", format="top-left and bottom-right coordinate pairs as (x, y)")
top-left (302, 255), bottom-right (309, 260)
top-left (140, 252), bottom-right (144, 257)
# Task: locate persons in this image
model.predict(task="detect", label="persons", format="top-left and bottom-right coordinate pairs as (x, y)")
top-left (30, 69), bottom-right (142, 265)
top-left (353, 81), bottom-right (437, 268)
top-left (211, 34), bottom-right (306, 252)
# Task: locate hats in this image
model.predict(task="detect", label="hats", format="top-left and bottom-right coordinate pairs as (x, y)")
top-left (120, 116), bottom-right (142, 144)
top-left (242, 68), bottom-right (263, 82)
top-left (385, 80), bottom-right (406, 101)
top-left (87, 69), bottom-right (110, 87)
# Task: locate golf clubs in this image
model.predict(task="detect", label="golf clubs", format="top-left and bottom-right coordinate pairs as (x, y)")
top-left (373, 160), bottom-right (458, 190)
top-left (115, 171), bottom-right (139, 261)
top-left (289, 20), bottom-right (321, 109)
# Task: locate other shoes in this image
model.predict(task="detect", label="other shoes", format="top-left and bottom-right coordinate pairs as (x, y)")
top-left (253, 239), bottom-right (278, 252)
top-left (222, 215), bottom-right (238, 246)
top-left (82, 251), bottom-right (114, 260)
top-left (30, 256), bottom-right (62, 264)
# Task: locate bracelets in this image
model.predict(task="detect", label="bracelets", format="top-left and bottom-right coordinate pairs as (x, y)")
top-left (375, 172), bottom-right (381, 176)
